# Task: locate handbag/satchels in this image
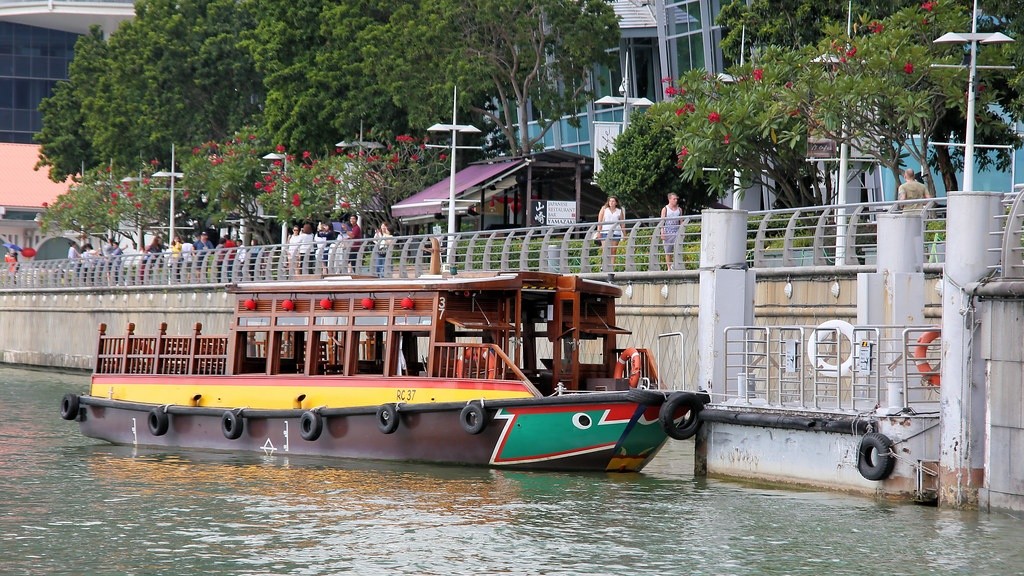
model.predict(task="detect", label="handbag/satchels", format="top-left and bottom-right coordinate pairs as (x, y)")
top-left (594, 240), bottom-right (602, 246)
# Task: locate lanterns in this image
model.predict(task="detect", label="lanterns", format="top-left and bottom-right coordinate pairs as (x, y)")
top-left (20, 248), bottom-right (38, 261)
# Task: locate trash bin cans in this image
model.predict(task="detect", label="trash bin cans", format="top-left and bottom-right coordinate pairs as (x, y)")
top-left (548, 245), bottom-right (561, 273)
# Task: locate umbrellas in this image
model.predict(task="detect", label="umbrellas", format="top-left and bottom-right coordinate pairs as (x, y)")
top-left (2, 243), bottom-right (24, 251)
top-left (331, 221), bottom-right (353, 232)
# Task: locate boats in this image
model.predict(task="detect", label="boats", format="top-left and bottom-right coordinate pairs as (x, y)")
top-left (60, 237), bottom-right (711, 473)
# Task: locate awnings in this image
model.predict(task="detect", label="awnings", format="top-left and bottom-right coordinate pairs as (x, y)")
top-left (389, 156), bottom-right (526, 224)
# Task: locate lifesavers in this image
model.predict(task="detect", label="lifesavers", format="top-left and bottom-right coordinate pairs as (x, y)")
top-left (459, 404), bottom-right (488, 435)
top-left (456, 347), bottom-right (496, 379)
top-left (855, 432), bottom-right (896, 481)
top-left (914, 331), bottom-right (941, 386)
top-left (60, 393), bottom-right (79, 420)
top-left (221, 410), bottom-right (244, 440)
top-left (659, 391), bottom-right (704, 440)
top-left (625, 387), bottom-right (664, 406)
top-left (298, 411), bottom-right (323, 441)
top-left (147, 408), bottom-right (169, 436)
top-left (118, 340), bottom-right (154, 364)
top-left (375, 404), bottom-right (400, 434)
top-left (613, 347), bottom-right (641, 388)
top-left (807, 319), bottom-right (856, 377)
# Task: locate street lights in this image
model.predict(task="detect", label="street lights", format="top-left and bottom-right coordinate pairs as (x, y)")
top-left (149, 143), bottom-right (195, 250)
top-left (929, 0), bottom-right (1015, 191)
top-left (260, 152), bottom-right (297, 245)
top-left (702, 25), bottom-right (768, 211)
top-left (71, 149), bottom-right (144, 253)
top-left (591, 51), bottom-right (656, 135)
top-left (424, 86), bottom-right (482, 265)
top-left (805, 1), bottom-right (880, 266)
top-left (333, 118), bottom-right (386, 238)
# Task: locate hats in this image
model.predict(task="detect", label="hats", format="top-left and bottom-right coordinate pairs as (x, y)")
top-left (201, 232), bottom-right (207, 235)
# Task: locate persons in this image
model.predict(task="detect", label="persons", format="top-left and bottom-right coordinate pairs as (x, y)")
top-left (341, 215), bottom-right (362, 273)
top-left (142, 231), bottom-right (215, 284)
top-left (373, 222), bottom-right (396, 278)
top-left (660, 193), bottom-right (684, 272)
top-left (214, 235), bottom-right (263, 284)
top-left (65, 239), bottom-right (128, 286)
top-left (5, 248), bottom-right (20, 285)
top-left (286, 221), bottom-right (336, 274)
top-left (898, 168), bottom-right (931, 214)
top-left (337, 232), bottom-right (351, 274)
top-left (597, 196), bottom-right (627, 271)
